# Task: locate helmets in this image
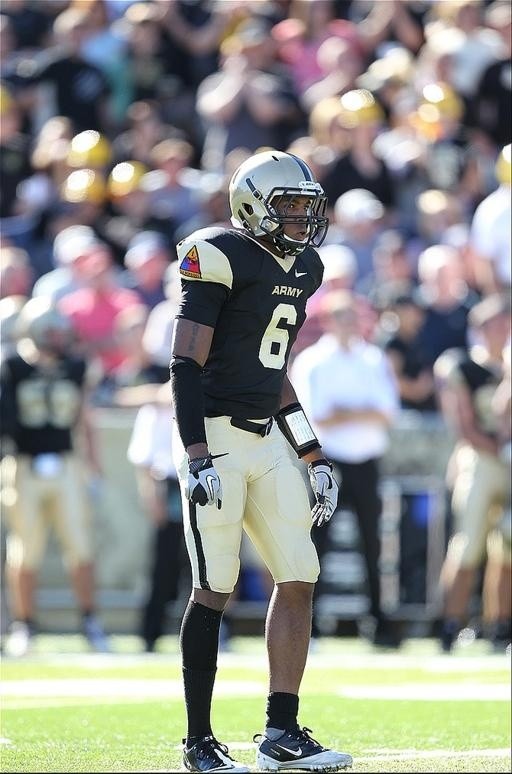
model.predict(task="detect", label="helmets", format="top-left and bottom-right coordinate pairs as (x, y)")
top-left (226, 148), bottom-right (326, 238)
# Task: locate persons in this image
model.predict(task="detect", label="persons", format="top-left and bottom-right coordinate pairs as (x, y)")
top-left (169, 151), bottom-right (355, 772)
top-left (67, 342), bottom-right (90, 349)
top-left (1, 1), bottom-right (512, 660)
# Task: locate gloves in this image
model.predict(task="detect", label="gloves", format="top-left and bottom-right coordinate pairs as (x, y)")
top-left (184, 452), bottom-right (225, 513)
top-left (308, 456), bottom-right (341, 530)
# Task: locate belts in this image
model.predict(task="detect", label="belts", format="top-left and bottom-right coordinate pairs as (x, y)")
top-left (229, 414), bottom-right (275, 439)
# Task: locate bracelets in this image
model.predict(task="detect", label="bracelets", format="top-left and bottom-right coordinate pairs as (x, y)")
top-left (272, 399), bottom-right (320, 460)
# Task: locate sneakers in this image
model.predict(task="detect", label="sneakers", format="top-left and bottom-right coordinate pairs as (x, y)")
top-left (255, 724), bottom-right (354, 773)
top-left (179, 732), bottom-right (252, 773)
